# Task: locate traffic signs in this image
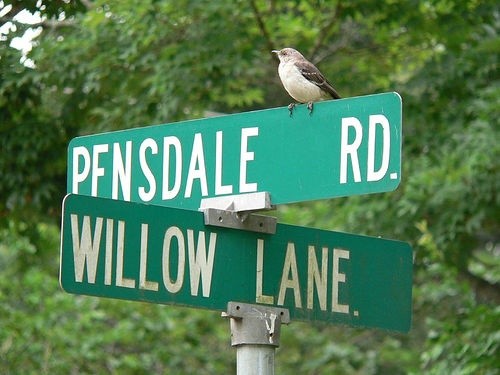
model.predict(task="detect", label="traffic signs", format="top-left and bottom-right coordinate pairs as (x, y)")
top-left (62, 90), bottom-right (414, 334)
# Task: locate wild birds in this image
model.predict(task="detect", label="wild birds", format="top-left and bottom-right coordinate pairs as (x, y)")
top-left (271, 47), bottom-right (341, 113)
